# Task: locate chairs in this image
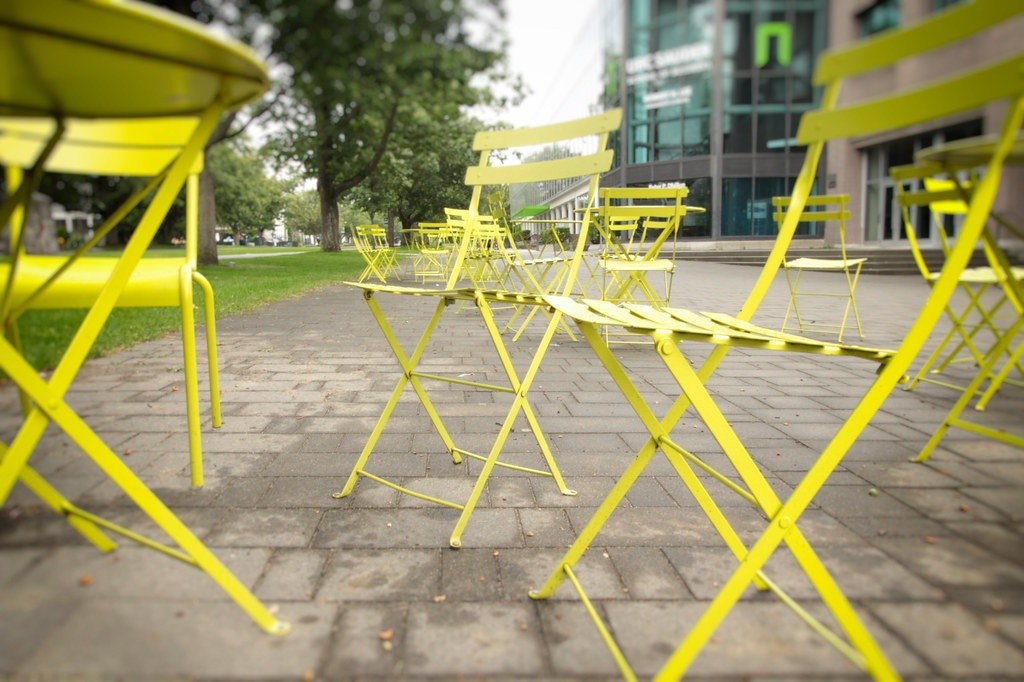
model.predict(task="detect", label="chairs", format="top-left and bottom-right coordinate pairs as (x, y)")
top-left (0, 0), bottom-right (1024, 682)
